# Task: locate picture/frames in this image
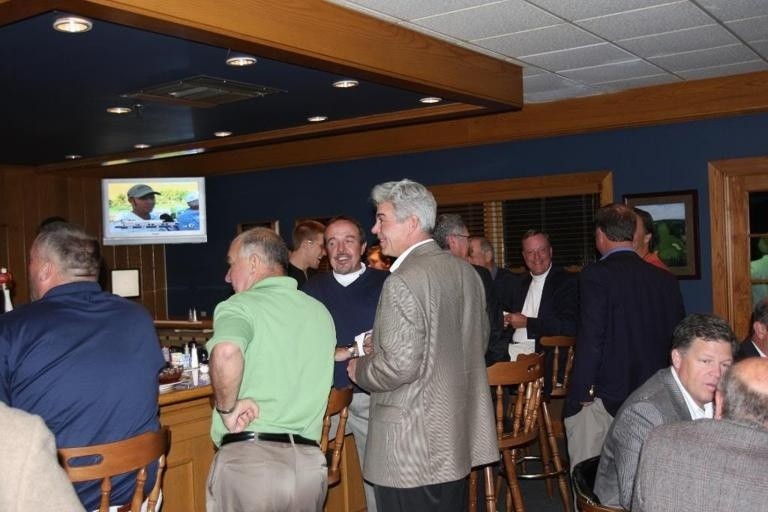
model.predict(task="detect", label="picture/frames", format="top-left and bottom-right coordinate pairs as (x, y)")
top-left (621, 188), bottom-right (700, 279)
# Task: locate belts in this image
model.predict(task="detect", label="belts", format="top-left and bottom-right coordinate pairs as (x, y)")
top-left (222, 431), bottom-right (319, 447)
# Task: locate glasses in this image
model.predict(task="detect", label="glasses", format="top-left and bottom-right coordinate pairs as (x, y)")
top-left (458, 235), bottom-right (472, 241)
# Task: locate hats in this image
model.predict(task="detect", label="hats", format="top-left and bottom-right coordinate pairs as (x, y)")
top-left (127, 183), bottom-right (160, 199)
top-left (186, 192), bottom-right (198, 202)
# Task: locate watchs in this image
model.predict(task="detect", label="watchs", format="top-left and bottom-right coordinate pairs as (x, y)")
top-left (215, 402), bottom-right (236, 413)
top-left (345, 344), bottom-right (357, 359)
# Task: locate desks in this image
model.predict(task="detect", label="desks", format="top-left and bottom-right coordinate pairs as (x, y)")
top-left (152, 317), bottom-right (370, 512)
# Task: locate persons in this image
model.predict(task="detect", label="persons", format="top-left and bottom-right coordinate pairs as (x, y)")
top-left (368, 246), bottom-right (392, 268)
top-left (0, 219), bottom-right (166, 511)
top-left (207, 230), bottom-right (338, 512)
top-left (632, 207), bottom-right (668, 270)
top-left (631, 356), bottom-right (767, 511)
top-left (733, 295), bottom-right (768, 358)
top-left (563, 204), bottom-right (683, 490)
top-left (592, 314), bottom-right (735, 510)
top-left (469, 236), bottom-right (499, 277)
top-left (0, 400), bottom-right (86, 512)
top-left (347, 179), bottom-right (499, 511)
top-left (318, 220), bottom-right (385, 512)
top-left (112, 184), bottom-right (167, 233)
top-left (497, 221), bottom-right (570, 364)
top-left (172, 193), bottom-right (199, 231)
top-left (436, 212), bottom-right (491, 312)
top-left (285, 223), bottom-right (324, 292)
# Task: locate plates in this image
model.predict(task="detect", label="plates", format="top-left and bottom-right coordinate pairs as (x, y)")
top-left (159, 378), bottom-right (188, 392)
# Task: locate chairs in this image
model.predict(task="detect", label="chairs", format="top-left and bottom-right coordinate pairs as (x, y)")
top-left (505, 337), bottom-right (577, 479)
top-left (320, 386), bottom-right (356, 486)
top-left (56, 426), bottom-right (176, 512)
top-left (469, 352), bottom-right (571, 512)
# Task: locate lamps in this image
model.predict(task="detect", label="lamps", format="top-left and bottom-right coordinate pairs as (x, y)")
top-left (53, 13), bottom-right (92, 34)
top-left (331, 74), bottom-right (359, 89)
top-left (107, 97), bottom-right (133, 113)
top-left (418, 94), bottom-right (442, 104)
top-left (226, 48), bottom-right (257, 67)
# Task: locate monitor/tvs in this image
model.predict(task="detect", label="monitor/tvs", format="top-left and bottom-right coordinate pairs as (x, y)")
top-left (100, 177), bottom-right (207, 246)
